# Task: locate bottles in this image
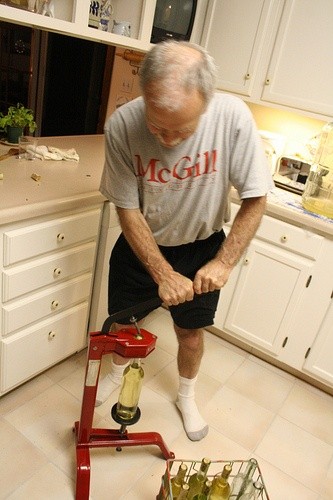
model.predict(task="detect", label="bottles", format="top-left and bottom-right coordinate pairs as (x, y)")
top-left (302, 121), bottom-right (333, 219)
top-left (185, 457), bottom-right (211, 500)
top-left (116, 357), bottom-right (144, 420)
top-left (208, 464), bottom-right (232, 500)
top-left (173, 483), bottom-right (190, 500)
top-left (170, 464), bottom-right (188, 499)
top-left (228, 458), bottom-right (257, 500)
top-left (192, 480), bottom-right (212, 500)
top-left (238, 481), bottom-right (263, 500)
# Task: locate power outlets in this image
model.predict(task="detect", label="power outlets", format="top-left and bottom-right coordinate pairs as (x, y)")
top-left (120, 78), bottom-right (134, 92)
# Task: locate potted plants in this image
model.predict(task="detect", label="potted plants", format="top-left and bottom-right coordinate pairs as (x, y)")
top-left (0, 104), bottom-right (37, 144)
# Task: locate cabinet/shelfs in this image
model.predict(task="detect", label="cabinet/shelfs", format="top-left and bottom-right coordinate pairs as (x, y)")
top-left (188, 196), bottom-right (321, 357)
top-left (0, 0), bottom-right (157, 53)
top-left (201, 0), bottom-right (333, 119)
top-left (303, 296), bottom-right (333, 388)
top-left (0, 204), bottom-right (104, 396)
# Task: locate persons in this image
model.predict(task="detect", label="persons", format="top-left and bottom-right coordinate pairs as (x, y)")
top-left (94, 43), bottom-right (275, 440)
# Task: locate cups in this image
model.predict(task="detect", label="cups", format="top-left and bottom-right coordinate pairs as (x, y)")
top-left (112, 20), bottom-right (132, 37)
top-left (19, 136), bottom-right (37, 161)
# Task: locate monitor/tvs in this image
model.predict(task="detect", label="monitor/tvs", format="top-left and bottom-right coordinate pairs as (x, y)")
top-left (150, 0), bottom-right (196, 44)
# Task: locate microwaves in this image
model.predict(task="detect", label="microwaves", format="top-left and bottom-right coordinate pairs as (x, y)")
top-left (272, 155), bottom-right (329, 196)
